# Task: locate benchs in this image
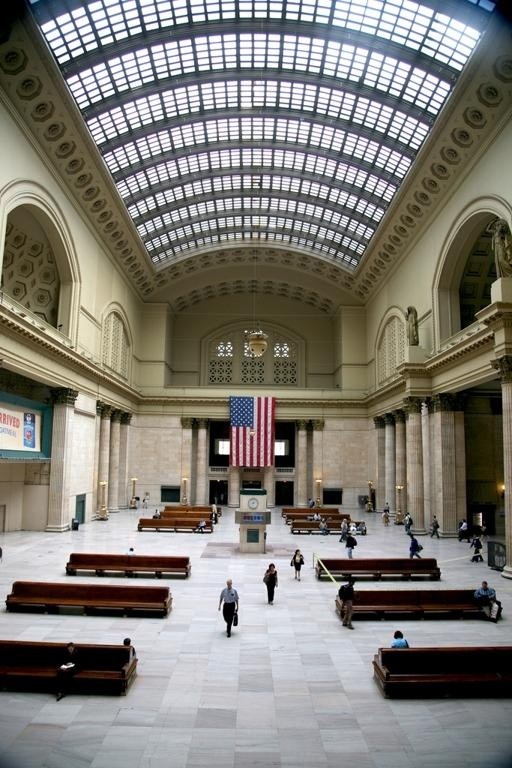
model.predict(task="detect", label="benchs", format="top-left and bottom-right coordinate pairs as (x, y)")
top-left (372, 646), bottom-right (512, 699)
top-left (335, 590), bottom-right (488, 620)
top-left (282, 507), bottom-right (365, 533)
top-left (6, 581), bottom-right (173, 617)
top-left (137, 506), bottom-right (221, 533)
top-left (0, 640), bottom-right (138, 696)
top-left (315, 558), bottom-right (441, 581)
top-left (64, 553), bottom-right (192, 579)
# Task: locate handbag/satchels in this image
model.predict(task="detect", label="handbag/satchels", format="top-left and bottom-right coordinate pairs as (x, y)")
top-left (233, 611), bottom-right (238, 626)
top-left (416, 545), bottom-right (423, 551)
top-left (263, 575), bottom-right (269, 583)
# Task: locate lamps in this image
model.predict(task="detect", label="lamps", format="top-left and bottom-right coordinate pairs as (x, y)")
top-left (99, 481), bottom-right (110, 520)
top-left (395, 486), bottom-right (403, 525)
top-left (245, 248), bottom-right (268, 358)
top-left (182, 478), bottom-right (188, 506)
top-left (315, 479), bottom-right (322, 507)
top-left (129, 478), bottom-right (139, 508)
top-left (366, 480), bottom-right (376, 512)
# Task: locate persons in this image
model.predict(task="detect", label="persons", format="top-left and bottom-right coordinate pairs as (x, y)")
top-left (264, 563), bottom-right (278, 604)
top-left (123, 637), bottom-right (135, 655)
top-left (140, 492), bottom-right (217, 530)
top-left (55, 642), bottom-right (78, 700)
top-left (218, 578), bottom-right (239, 636)
top-left (390, 630), bottom-right (409, 647)
top-left (126, 547), bottom-right (134, 555)
top-left (291, 496), bottom-right (500, 630)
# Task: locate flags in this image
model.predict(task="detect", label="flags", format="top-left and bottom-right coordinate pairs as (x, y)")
top-left (229, 395), bottom-right (274, 467)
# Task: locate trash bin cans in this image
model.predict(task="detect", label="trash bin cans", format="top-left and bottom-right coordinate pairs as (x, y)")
top-left (72, 518), bottom-right (79, 531)
top-left (365, 500), bottom-right (373, 512)
top-left (486, 541), bottom-right (507, 572)
